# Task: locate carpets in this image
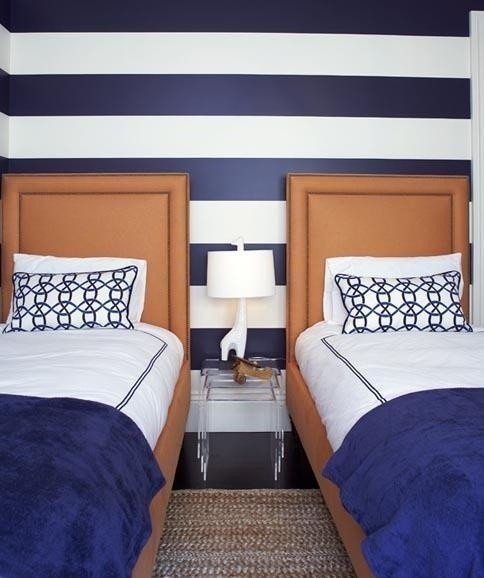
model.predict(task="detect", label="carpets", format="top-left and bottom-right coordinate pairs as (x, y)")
top-left (153, 487), bottom-right (358, 578)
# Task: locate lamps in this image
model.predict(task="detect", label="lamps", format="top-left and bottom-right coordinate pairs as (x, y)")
top-left (205, 236), bottom-right (276, 362)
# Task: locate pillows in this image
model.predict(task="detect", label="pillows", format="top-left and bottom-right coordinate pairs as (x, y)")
top-left (334, 270), bottom-right (473, 334)
top-left (320, 252), bottom-right (465, 324)
top-left (7, 253), bottom-right (149, 324)
top-left (1, 266), bottom-right (139, 332)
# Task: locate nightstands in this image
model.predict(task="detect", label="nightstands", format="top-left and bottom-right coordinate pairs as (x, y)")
top-left (196, 356), bottom-right (285, 483)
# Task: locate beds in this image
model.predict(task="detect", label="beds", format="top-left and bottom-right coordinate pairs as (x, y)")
top-left (286, 172), bottom-right (482, 578)
top-left (0, 172), bottom-right (193, 576)
